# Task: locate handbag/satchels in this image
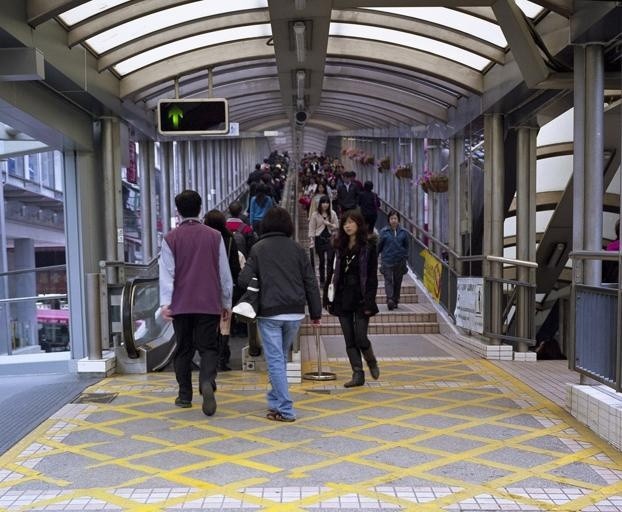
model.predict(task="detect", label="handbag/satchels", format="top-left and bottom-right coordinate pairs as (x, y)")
top-left (299, 196), bottom-right (311, 206)
top-left (327, 284), bottom-right (335, 303)
top-left (232, 274), bottom-right (260, 324)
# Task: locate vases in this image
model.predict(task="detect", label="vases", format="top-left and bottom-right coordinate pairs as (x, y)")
top-left (421, 180), bottom-right (435, 193)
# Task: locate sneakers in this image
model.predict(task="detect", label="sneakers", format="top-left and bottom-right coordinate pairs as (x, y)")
top-left (175, 397), bottom-right (192, 408)
top-left (387, 298), bottom-right (398, 310)
top-left (266, 410), bottom-right (296, 421)
top-left (201, 380), bottom-right (217, 416)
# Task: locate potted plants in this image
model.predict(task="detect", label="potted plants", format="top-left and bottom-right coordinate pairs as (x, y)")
top-left (434, 174), bottom-right (447, 191)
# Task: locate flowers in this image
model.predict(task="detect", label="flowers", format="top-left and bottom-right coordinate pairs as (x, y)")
top-left (342, 148), bottom-right (412, 174)
top-left (415, 170), bottom-right (431, 184)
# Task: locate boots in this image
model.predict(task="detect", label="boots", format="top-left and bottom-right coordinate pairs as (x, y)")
top-left (344, 342), bottom-right (379, 388)
top-left (216, 333), bottom-right (231, 371)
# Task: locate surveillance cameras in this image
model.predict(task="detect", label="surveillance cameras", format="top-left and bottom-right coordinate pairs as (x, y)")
top-left (294, 106), bottom-right (309, 123)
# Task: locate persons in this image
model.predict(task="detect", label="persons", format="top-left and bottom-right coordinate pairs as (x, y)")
top-left (203, 210), bottom-right (242, 371)
top-left (238, 208), bottom-right (322, 421)
top-left (378, 211), bottom-right (410, 310)
top-left (230, 231), bottom-right (247, 336)
top-left (360, 180), bottom-right (381, 234)
top-left (248, 185), bottom-right (273, 235)
top-left (160, 191), bottom-right (233, 416)
top-left (322, 210), bottom-right (379, 388)
top-left (247, 150), bottom-right (289, 206)
top-left (301, 152), bottom-right (360, 215)
top-left (309, 196), bottom-right (339, 289)
top-left (226, 202), bottom-right (253, 255)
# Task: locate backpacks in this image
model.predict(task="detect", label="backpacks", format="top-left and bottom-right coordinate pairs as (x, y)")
top-left (225, 223), bottom-right (246, 254)
